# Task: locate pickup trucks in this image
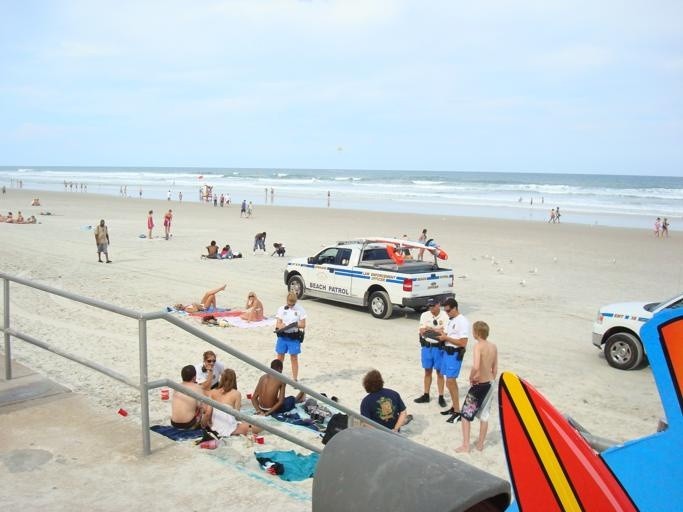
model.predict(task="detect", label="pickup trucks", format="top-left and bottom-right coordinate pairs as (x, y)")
top-left (283, 240), bottom-right (456, 320)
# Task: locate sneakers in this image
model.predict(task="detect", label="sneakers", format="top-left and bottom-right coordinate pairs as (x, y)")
top-left (439, 395), bottom-right (447, 407)
top-left (439, 407), bottom-right (454, 415)
top-left (413, 394), bottom-right (429, 403)
top-left (446, 412), bottom-right (461, 423)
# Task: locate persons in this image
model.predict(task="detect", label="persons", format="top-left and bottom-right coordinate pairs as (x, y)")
top-left (169, 365), bottom-right (206, 431)
top-left (412, 297), bottom-right (446, 407)
top-left (652, 217), bottom-right (660, 237)
top-left (1, 179), bottom-right (41, 224)
top-left (661, 217), bottom-right (670, 238)
top-left (253, 232), bottom-right (285, 256)
top-left (120, 182), bottom-right (274, 219)
top-left (402, 235), bottom-right (410, 255)
top-left (454, 321), bottom-right (498, 453)
top-left (419, 298), bottom-right (469, 423)
top-left (147, 210), bottom-right (173, 239)
top-left (204, 367), bottom-right (262, 434)
top-left (249, 361), bottom-right (306, 417)
top-left (274, 292), bottom-right (306, 389)
top-left (172, 283), bottom-right (228, 311)
top-left (64, 179), bottom-right (88, 191)
top-left (94, 220), bottom-right (112, 263)
top-left (196, 351), bottom-right (225, 391)
top-left (547, 208), bottom-right (555, 223)
top-left (205, 240), bottom-right (242, 258)
top-left (554, 207), bottom-right (561, 223)
top-left (239, 291), bottom-right (263, 324)
top-left (416, 229), bottom-right (427, 261)
top-left (352, 368), bottom-right (406, 433)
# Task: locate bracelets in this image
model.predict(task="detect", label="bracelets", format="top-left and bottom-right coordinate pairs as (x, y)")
top-left (256, 409), bottom-right (261, 413)
top-left (394, 428), bottom-right (398, 431)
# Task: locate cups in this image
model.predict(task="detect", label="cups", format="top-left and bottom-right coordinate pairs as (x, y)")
top-left (161, 388), bottom-right (168, 400)
top-left (255, 436), bottom-right (264, 444)
top-left (117, 408), bottom-right (127, 416)
top-left (199, 439), bottom-right (216, 449)
top-left (246, 393), bottom-right (252, 398)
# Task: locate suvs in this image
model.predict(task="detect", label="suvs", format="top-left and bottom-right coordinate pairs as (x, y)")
top-left (591, 289), bottom-right (683, 372)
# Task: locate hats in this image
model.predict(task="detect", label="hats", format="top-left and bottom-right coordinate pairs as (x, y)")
top-left (426, 296), bottom-right (440, 306)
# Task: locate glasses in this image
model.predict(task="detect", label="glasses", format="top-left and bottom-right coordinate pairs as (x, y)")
top-left (206, 360), bottom-right (216, 363)
top-left (446, 308), bottom-right (452, 313)
